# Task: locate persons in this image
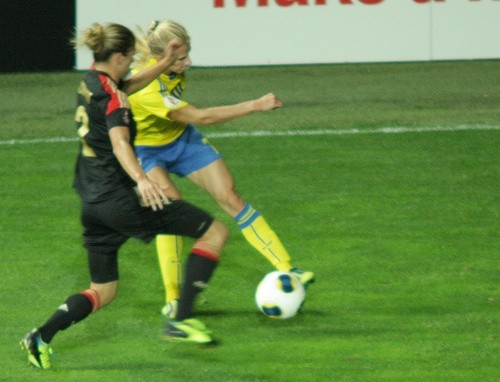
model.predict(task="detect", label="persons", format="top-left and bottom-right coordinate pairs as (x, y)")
top-left (19, 22), bottom-right (228, 371)
top-left (122, 19), bottom-right (316, 320)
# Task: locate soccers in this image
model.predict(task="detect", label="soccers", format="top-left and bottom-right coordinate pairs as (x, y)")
top-left (256, 271), bottom-right (305, 319)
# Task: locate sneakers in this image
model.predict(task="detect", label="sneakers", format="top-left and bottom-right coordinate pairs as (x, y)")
top-left (158, 320), bottom-right (212, 343)
top-left (162, 299), bottom-right (181, 319)
top-left (19, 324), bottom-right (52, 369)
top-left (288, 268), bottom-right (315, 290)
